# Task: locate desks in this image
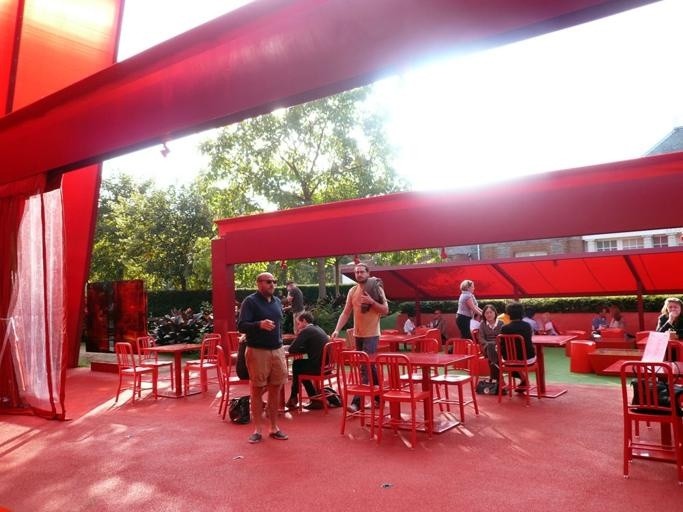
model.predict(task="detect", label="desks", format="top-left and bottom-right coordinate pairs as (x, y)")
top-left (142, 343), bottom-right (204, 398)
top-left (603, 359), bottom-right (683, 453)
top-left (530, 335), bottom-right (577, 398)
top-left (587, 347), bottom-right (645, 376)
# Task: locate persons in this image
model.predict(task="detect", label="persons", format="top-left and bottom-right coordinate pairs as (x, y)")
top-left (285, 312), bottom-right (330, 409)
top-left (479, 305), bottom-right (506, 396)
top-left (283, 281), bottom-right (305, 335)
top-left (655, 298), bottom-right (683, 361)
top-left (455, 279), bottom-right (483, 340)
top-left (404, 306), bottom-right (446, 344)
top-left (237, 273), bottom-right (289, 443)
top-left (198, 303), bottom-right (239, 335)
top-left (591, 306), bottom-right (625, 340)
top-left (470, 307), bottom-right (556, 342)
top-left (236, 334), bottom-right (273, 410)
top-left (499, 302), bottom-right (536, 392)
top-left (330, 263), bottom-right (389, 413)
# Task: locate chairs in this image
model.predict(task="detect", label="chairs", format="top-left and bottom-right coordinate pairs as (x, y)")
top-left (620, 362), bottom-right (683, 485)
top-left (496, 335), bottom-right (539, 403)
top-left (664, 341), bottom-right (683, 361)
top-left (566, 330), bottom-right (596, 374)
top-left (115, 342), bottom-right (158, 402)
top-left (137, 337), bottom-right (174, 400)
top-left (601, 327), bottom-right (625, 339)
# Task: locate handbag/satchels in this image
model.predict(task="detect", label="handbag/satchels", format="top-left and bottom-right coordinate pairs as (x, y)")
top-left (324, 387), bottom-right (341, 406)
top-left (477, 381), bottom-right (497, 395)
top-left (630, 378), bottom-right (683, 417)
top-left (229, 396), bottom-right (250, 423)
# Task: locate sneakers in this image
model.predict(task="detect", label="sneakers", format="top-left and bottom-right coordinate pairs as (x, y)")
top-left (346, 400), bottom-right (379, 413)
top-left (490, 362), bottom-right (528, 395)
top-left (248, 433), bottom-right (262, 443)
top-left (269, 429), bottom-right (288, 440)
top-left (286, 396), bottom-right (322, 408)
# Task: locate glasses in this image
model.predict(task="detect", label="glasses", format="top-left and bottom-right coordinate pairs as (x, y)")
top-left (261, 280), bottom-right (277, 284)
